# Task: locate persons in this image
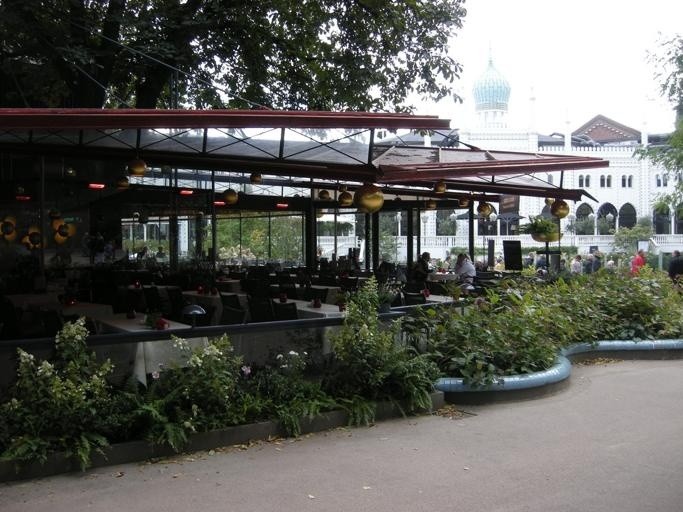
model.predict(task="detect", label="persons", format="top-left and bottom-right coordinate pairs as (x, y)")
top-left (136, 246), bottom-right (149, 258)
top-left (494, 247), bottom-right (682, 287)
top-left (427, 256), bottom-right (451, 271)
top-left (453, 252), bottom-right (476, 285)
top-left (410, 251), bottom-right (433, 279)
top-left (374, 252), bottom-right (408, 291)
top-left (153, 245), bottom-right (169, 258)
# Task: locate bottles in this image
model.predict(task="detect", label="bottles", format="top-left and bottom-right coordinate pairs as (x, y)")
top-left (125, 305), bottom-right (136, 318)
top-left (437, 266), bottom-right (453, 274)
top-left (307, 297), bottom-right (321, 308)
top-left (279, 293), bottom-right (287, 303)
top-left (67, 296), bottom-right (76, 305)
top-left (134, 279), bottom-right (140, 288)
top-left (419, 289), bottom-right (430, 298)
top-left (197, 285), bottom-right (203, 295)
